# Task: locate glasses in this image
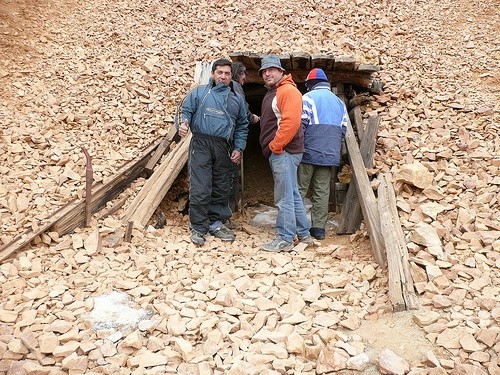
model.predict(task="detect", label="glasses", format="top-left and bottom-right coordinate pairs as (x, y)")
top-left (305, 82), bottom-right (308, 87)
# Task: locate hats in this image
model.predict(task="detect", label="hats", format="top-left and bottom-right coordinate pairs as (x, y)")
top-left (258, 55), bottom-right (286, 76)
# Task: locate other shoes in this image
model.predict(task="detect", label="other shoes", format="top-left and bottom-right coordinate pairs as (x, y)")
top-left (309, 227), bottom-right (325, 240)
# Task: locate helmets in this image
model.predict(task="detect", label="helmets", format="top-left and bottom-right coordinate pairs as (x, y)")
top-left (306, 68), bottom-right (328, 82)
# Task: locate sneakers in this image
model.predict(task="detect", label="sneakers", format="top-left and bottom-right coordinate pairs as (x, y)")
top-left (210, 228), bottom-right (235, 241)
top-left (189, 229), bottom-right (204, 244)
top-left (261, 237), bottom-right (294, 252)
top-left (299, 236), bottom-right (314, 247)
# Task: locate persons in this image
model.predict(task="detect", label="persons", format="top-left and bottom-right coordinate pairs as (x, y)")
top-left (298, 68), bottom-right (347, 239)
top-left (259, 55), bottom-right (310, 253)
top-left (175, 59), bottom-right (249, 244)
top-left (229, 64), bottom-right (260, 213)
